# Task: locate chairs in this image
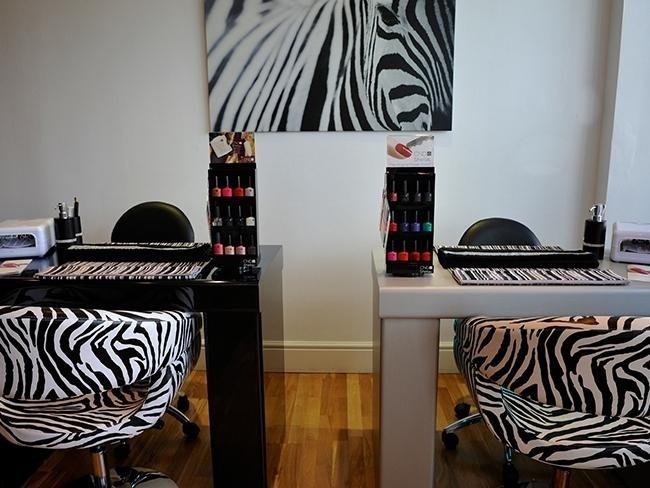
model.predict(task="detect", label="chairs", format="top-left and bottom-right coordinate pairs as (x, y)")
top-left (1, 301), bottom-right (202, 488)
top-left (453, 315), bottom-right (650, 488)
top-left (110, 199), bottom-right (201, 440)
top-left (441, 216), bottom-right (543, 486)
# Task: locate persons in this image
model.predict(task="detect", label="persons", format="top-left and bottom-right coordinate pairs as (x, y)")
top-left (387, 135), bottom-right (412, 161)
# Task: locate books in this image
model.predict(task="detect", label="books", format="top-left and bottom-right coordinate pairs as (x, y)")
top-left (0, 258), bottom-right (33, 276)
top-left (376, 195), bottom-right (392, 250)
top-left (625, 263), bottom-right (650, 286)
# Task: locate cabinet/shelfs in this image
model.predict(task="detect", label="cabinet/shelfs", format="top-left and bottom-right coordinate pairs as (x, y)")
top-left (384, 166), bottom-right (436, 276)
top-left (207, 161), bottom-right (260, 264)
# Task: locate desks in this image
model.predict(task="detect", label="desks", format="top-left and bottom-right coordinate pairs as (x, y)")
top-left (371, 245), bottom-right (650, 486)
top-left (2, 240), bottom-right (286, 486)
